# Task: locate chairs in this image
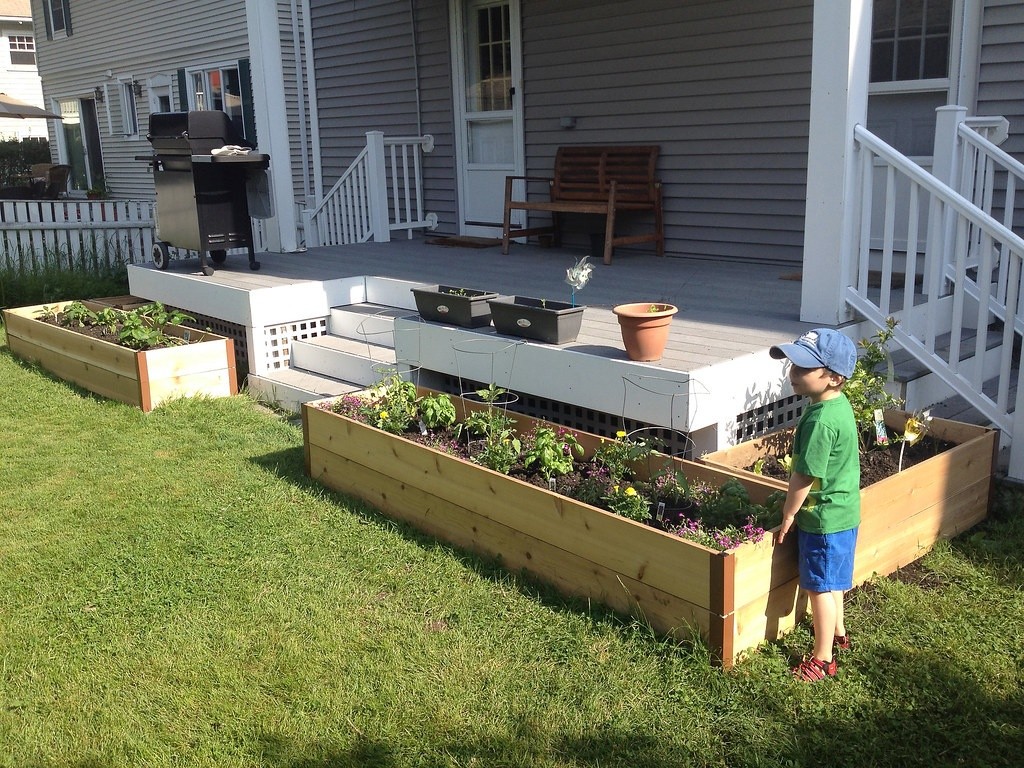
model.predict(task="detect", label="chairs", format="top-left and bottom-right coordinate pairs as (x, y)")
top-left (0, 162), bottom-right (72, 200)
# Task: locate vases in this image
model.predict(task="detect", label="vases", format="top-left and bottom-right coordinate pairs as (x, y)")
top-left (612, 302), bottom-right (678, 364)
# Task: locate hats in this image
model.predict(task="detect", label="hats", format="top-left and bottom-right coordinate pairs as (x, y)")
top-left (769, 328), bottom-right (857, 379)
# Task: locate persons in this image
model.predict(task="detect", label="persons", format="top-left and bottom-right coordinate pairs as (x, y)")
top-left (769, 328), bottom-right (861, 683)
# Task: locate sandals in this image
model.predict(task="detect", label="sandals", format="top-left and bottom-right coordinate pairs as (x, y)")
top-left (791, 658), bottom-right (836, 682)
top-left (833, 636), bottom-right (850, 649)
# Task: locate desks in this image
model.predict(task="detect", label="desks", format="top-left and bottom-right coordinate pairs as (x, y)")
top-left (9, 171), bottom-right (46, 184)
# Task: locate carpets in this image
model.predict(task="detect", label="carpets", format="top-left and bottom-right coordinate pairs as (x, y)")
top-left (424, 235), bottom-right (515, 249)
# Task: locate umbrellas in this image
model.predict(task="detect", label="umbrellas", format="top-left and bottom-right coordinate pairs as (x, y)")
top-left (0, 93), bottom-right (64, 119)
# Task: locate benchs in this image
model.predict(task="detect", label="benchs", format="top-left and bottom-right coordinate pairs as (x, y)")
top-left (499, 143), bottom-right (665, 263)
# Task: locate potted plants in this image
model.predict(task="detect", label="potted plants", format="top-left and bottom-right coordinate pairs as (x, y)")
top-left (84, 173), bottom-right (112, 199)
top-left (409, 284), bottom-right (585, 345)
